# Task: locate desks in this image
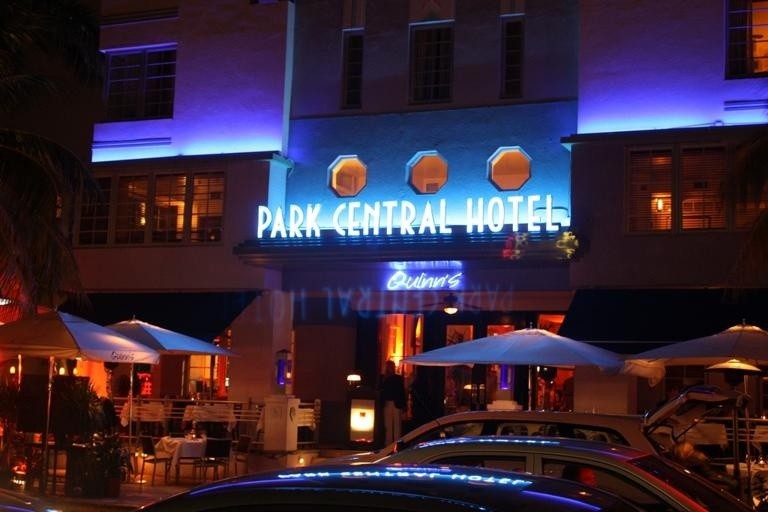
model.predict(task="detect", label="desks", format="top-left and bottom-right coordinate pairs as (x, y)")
top-left (120, 397), bottom-right (314, 454)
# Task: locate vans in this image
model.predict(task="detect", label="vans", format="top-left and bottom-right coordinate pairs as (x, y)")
top-left (310, 385), bottom-right (742, 482)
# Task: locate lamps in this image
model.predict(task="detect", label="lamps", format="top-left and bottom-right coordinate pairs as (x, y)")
top-left (442, 293), bottom-right (460, 315)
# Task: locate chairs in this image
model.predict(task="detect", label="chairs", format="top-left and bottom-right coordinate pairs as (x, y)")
top-left (139, 436), bottom-right (233, 487)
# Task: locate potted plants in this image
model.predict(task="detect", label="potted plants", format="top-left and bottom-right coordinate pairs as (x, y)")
top-left (97, 437), bottom-right (128, 499)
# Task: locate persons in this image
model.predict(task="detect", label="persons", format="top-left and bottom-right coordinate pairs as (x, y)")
top-left (375, 360), bottom-right (407, 448)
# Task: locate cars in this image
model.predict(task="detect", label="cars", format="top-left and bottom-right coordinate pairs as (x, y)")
top-left (306, 433), bottom-right (759, 512)
top-left (130, 459), bottom-right (654, 512)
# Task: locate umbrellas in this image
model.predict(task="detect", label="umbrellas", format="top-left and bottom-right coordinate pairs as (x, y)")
top-left (403, 318), bottom-right (668, 420)
top-left (0, 306), bottom-right (159, 491)
top-left (620, 321), bottom-right (768, 497)
top-left (102, 313), bottom-right (239, 453)
